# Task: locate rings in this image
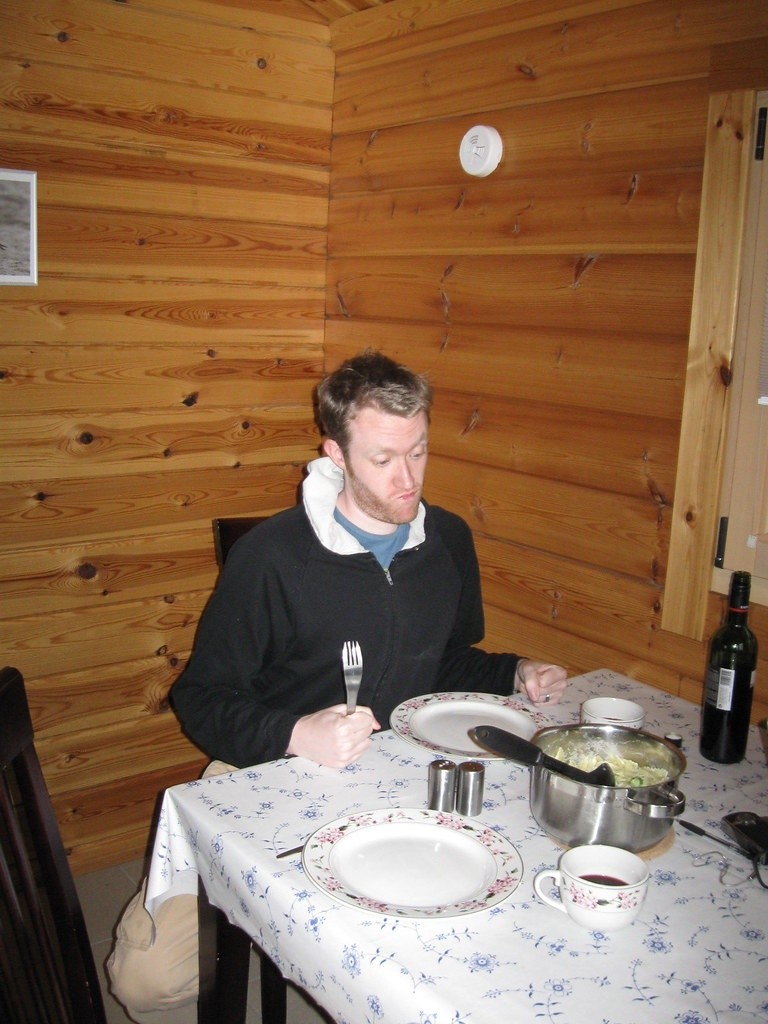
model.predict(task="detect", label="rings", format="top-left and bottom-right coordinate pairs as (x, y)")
top-left (545, 694), bottom-right (550, 702)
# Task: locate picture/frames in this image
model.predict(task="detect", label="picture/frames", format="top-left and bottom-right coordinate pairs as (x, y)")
top-left (0, 167), bottom-right (39, 287)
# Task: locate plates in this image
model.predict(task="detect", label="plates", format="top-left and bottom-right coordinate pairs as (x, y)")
top-left (302, 807), bottom-right (525, 920)
top-left (388, 691), bottom-right (556, 762)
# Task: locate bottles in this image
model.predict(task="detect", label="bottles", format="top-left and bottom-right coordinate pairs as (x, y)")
top-left (699, 570), bottom-right (759, 764)
top-left (427, 760), bottom-right (485, 817)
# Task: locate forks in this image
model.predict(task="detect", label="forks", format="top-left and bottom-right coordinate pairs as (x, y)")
top-left (338, 640), bottom-right (364, 717)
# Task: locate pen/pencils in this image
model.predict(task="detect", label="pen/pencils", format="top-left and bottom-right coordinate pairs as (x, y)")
top-left (676, 819), bottom-right (761, 864)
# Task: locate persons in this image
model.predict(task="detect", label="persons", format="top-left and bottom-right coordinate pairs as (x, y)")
top-left (103, 353), bottom-right (567, 1024)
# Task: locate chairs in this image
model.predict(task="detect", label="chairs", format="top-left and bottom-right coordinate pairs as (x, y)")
top-left (0, 665), bottom-right (110, 1024)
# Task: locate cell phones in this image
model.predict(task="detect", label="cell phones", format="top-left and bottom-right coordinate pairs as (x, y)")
top-left (720, 812), bottom-right (768, 865)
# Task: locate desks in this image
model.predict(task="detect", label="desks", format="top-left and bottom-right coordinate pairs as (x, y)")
top-left (143, 667), bottom-right (768, 1024)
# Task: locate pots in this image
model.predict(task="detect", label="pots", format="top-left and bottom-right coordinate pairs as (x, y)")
top-left (527, 722), bottom-right (688, 854)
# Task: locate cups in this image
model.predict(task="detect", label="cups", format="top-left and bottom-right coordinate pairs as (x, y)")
top-left (580, 697), bottom-right (647, 732)
top-left (534, 843), bottom-right (651, 933)
